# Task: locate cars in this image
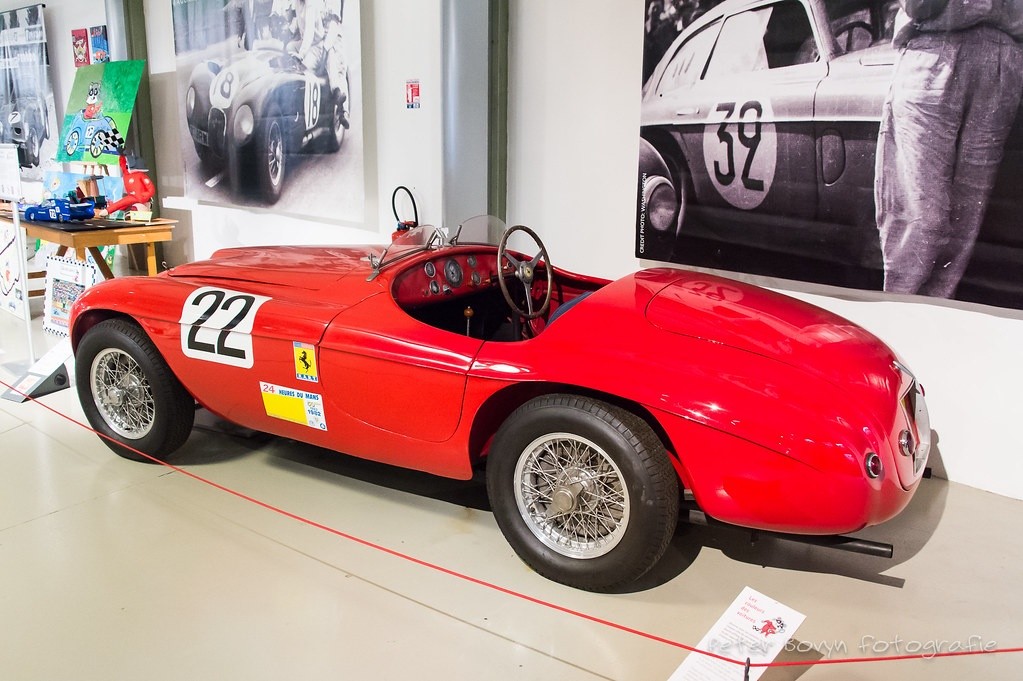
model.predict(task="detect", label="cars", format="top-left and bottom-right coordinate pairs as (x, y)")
top-left (187, 33), bottom-right (353, 203)
top-left (0, 75), bottom-right (50, 169)
top-left (637, 0), bottom-right (1023, 295)
top-left (24, 198), bottom-right (95, 224)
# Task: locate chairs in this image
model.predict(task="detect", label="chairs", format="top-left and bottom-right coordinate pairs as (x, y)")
top-left (544, 288), bottom-right (593, 325)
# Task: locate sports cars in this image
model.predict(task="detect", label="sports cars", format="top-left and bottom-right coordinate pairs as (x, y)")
top-left (67, 184), bottom-right (935, 595)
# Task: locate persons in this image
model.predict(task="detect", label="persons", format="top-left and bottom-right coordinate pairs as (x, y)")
top-left (237, 0), bottom-right (350, 129)
top-left (873, 0), bottom-right (1023, 301)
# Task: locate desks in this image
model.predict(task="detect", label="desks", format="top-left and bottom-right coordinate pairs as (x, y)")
top-left (1, 213), bottom-right (177, 279)
